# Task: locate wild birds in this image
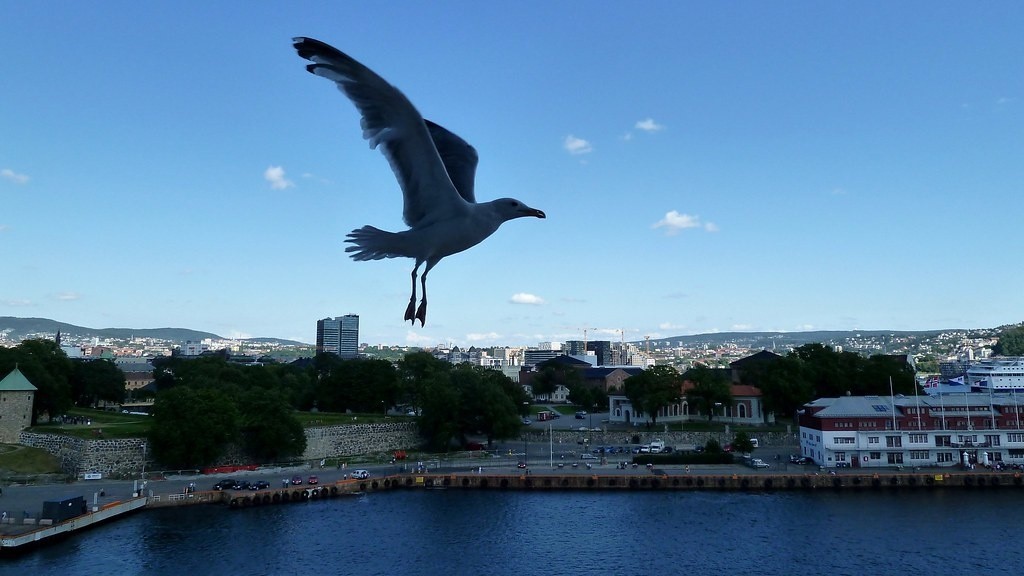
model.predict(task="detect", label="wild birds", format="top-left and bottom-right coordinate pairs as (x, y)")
top-left (292, 36), bottom-right (547, 329)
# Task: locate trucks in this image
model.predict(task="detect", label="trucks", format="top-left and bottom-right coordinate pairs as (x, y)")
top-left (536, 410), bottom-right (561, 422)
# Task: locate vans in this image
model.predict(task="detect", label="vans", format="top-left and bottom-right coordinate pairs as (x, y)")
top-left (575, 412), bottom-right (586, 419)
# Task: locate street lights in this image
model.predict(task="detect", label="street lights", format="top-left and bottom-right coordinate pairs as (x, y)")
top-left (715, 402), bottom-right (735, 439)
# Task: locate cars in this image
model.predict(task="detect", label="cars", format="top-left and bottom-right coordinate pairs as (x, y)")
top-left (291, 473), bottom-right (302, 485)
top-left (753, 459), bottom-right (763, 462)
top-left (351, 470), bottom-right (368, 480)
top-left (695, 447), bottom-right (703, 453)
top-left (309, 474), bottom-right (318, 485)
top-left (523, 420), bottom-right (532, 424)
top-left (742, 451), bottom-right (750, 460)
top-left (213, 479), bottom-right (236, 490)
top-left (594, 440), bottom-right (672, 454)
top-left (517, 461), bottom-right (526, 469)
top-left (247, 481), bottom-right (270, 491)
top-left (749, 439), bottom-right (759, 448)
top-left (232, 480), bottom-right (250, 491)
top-left (791, 456), bottom-right (814, 465)
top-left (752, 461), bottom-right (770, 469)
top-left (722, 444), bottom-right (733, 452)
